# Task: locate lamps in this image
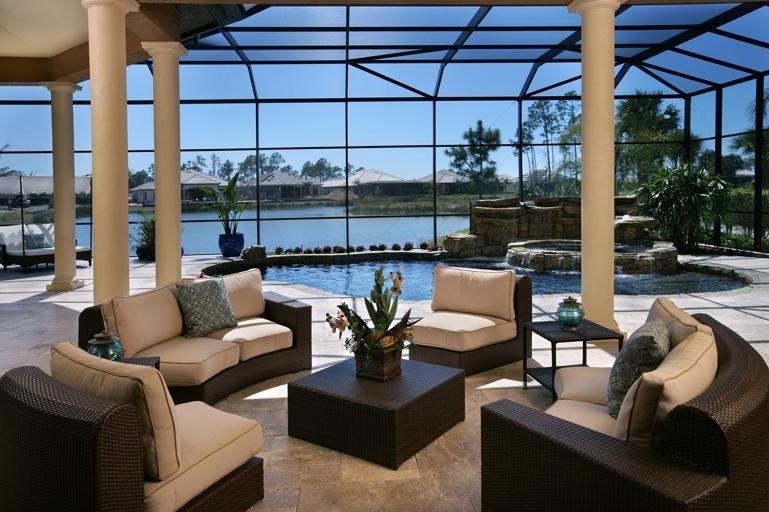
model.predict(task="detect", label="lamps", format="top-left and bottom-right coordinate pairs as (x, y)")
top-left (555, 296), bottom-right (584, 331)
top-left (88, 331), bottom-right (124, 363)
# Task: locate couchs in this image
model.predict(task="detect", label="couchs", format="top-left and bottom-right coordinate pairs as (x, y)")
top-left (408, 265), bottom-right (533, 377)
top-left (0, 223), bottom-right (93, 271)
top-left (0, 340), bottom-right (265, 512)
top-left (77, 267), bottom-right (312, 406)
top-left (480, 296), bottom-right (769, 512)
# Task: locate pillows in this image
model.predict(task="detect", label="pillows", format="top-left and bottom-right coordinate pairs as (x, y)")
top-left (24, 233), bottom-right (53, 249)
top-left (607, 318), bottom-right (671, 421)
top-left (175, 275), bottom-right (238, 339)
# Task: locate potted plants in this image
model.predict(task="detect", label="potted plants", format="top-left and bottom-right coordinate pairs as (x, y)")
top-left (193, 172), bottom-right (255, 257)
top-left (135, 209), bottom-right (155, 262)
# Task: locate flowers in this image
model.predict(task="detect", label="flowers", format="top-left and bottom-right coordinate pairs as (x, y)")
top-left (326, 266), bottom-right (424, 352)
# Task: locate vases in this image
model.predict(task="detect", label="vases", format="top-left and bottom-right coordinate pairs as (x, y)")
top-left (355, 347), bottom-right (402, 383)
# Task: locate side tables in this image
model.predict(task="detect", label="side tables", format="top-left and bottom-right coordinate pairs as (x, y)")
top-left (121, 356), bottom-right (160, 371)
top-left (522, 319), bottom-right (624, 405)
